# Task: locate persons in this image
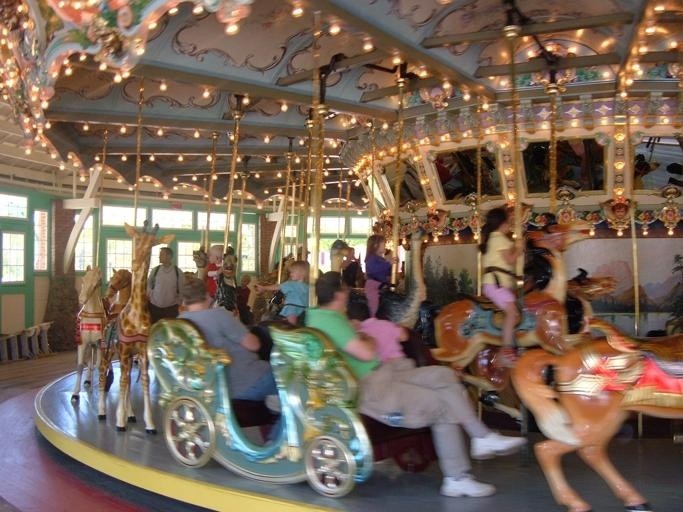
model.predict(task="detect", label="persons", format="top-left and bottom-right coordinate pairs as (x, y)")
top-left (254, 247), bottom-right (311, 327)
top-left (180, 279), bottom-right (284, 446)
top-left (477, 209), bottom-right (527, 369)
top-left (343, 234), bottom-right (407, 365)
top-left (306, 271), bottom-right (528, 497)
top-left (207, 255), bottom-right (251, 323)
top-left (147, 248), bottom-right (187, 323)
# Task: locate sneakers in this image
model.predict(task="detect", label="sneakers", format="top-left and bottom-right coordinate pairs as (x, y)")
top-left (494, 347), bottom-right (516, 368)
top-left (264, 394), bottom-right (281, 413)
top-left (440, 475), bottom-right (494, 498)
top-left (470, 432), bottom-right (528, 460)
